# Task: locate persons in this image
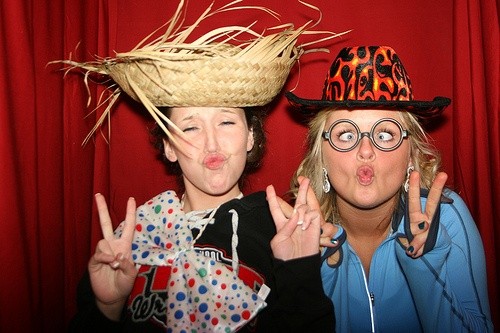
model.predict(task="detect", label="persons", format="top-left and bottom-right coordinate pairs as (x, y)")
top-left (276, 45), bottom-right (494, 333)
top-left (73, 49), bottom-right (335, 333)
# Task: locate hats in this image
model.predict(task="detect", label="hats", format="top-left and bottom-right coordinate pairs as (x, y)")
top-left (46, 0), bottom-right (353, 159)
top-left (286, 46), bottom-right (451, 113)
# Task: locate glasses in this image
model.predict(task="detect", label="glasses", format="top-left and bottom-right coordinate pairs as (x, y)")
top-left (322, 118), bottom-right (409, 151)
